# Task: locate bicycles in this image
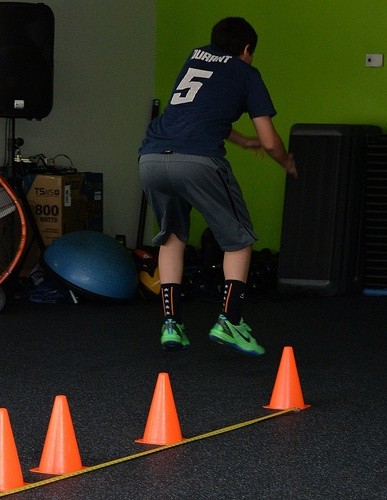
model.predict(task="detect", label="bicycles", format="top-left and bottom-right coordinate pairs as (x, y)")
top-left (0, 169), bottom-right (37, 282)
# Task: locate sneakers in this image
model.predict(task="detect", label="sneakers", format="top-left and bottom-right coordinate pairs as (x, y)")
top-left (161, 319), bottom-right (192, 350)
top-left (209, 313), bottom-right (265, 356)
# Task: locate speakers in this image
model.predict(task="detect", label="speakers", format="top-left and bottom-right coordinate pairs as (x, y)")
top-left (0, 3), bottom-right (55, 120)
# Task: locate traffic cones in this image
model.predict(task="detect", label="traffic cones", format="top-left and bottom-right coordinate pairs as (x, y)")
top-left (134, 371), bottom-right (193, 448)
top-left (30, 394), bottom-right (91, 475)
top-left (262, 343), bottom-right (313, 414)
top-left (0, 407), bottom-right (30, 492)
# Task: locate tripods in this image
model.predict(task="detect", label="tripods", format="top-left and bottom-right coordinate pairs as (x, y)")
top-left (0, 118), bottom-right (78, 305)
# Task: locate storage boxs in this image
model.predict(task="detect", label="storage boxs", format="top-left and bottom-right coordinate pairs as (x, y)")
top-left (0, 171), bottom-right (104, 281)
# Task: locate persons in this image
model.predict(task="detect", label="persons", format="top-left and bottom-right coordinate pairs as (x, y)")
top-left (139, 15), bottom-right (298, 356)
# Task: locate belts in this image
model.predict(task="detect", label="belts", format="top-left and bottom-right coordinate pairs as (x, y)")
top-left (160, 150), bottom-right (172, 154)
